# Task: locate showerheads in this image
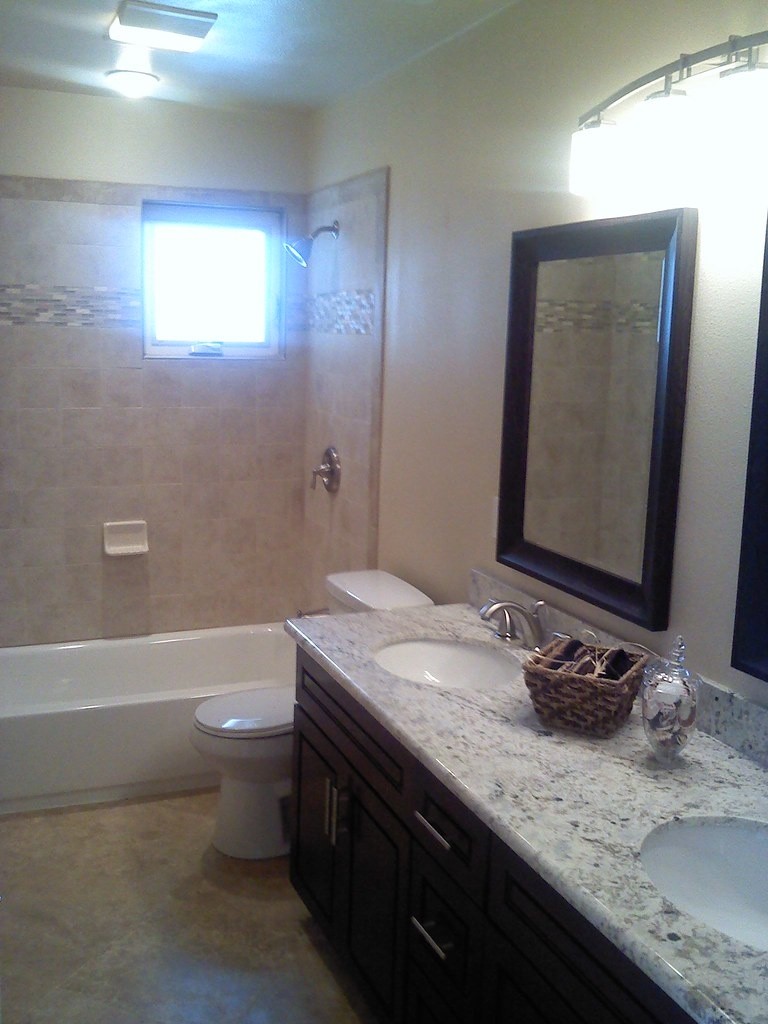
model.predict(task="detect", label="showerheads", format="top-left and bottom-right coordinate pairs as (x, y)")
top-left (283, 220), bottom-right (340, 268)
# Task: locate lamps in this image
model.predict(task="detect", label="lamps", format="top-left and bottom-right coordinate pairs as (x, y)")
top-left (108, 0), bottom-right (218, 53)
top-left (570, 30), bottom-right (768, 168)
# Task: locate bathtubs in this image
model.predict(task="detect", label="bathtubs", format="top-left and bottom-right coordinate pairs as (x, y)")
top-left (0, 615), bottom-right (330, 817)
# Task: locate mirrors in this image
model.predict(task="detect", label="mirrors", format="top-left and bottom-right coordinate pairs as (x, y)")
top-left (495, 208), bottom-right (699, 631)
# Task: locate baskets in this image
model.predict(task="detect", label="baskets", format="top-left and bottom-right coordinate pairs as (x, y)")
top-left (522, 637), bottom-right (648, 738)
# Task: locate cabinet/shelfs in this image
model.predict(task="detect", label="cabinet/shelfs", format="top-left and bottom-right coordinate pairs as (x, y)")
top-left (289, 643), bottom-right (697, 1024)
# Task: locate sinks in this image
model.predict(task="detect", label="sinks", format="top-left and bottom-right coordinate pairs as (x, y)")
top-left (634, 811), bottom-right (768, 949)
top-left (368, 631), bottom-right (523, 689)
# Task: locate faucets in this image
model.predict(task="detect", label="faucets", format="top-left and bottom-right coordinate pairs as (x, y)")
top-left (478, 598), bottom-right (545, 650)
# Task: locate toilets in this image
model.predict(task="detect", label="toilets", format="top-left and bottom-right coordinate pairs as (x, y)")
top-left (185, 569), bottom-right (437, 861)
